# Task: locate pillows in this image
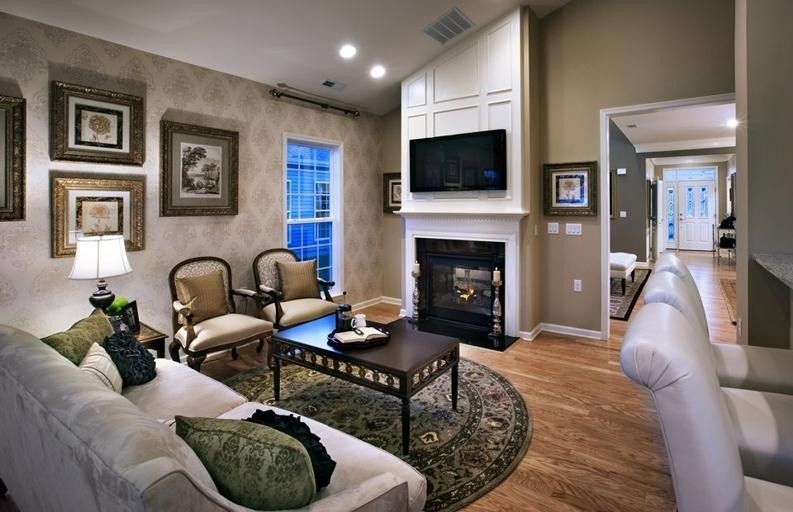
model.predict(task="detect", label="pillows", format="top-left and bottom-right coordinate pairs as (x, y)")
top-left (276, 257), bottom-right (318, 298)
top-left (174, 414), bottom-right (315, 510)
top-left (40, 306), bottom-right (115, 367)
top-left (80, 341), bottom-right (123, 397)
top-left (174, 270), bottom-right (228, 324)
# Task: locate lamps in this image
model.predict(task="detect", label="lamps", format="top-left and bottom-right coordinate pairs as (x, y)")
top-left (68, 234), bottom-right (132, 315)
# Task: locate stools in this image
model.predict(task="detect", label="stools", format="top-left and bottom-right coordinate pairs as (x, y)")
top-left (610, 252), bottom-right (637, 295)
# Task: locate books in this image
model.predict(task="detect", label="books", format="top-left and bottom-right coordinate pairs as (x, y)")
top-left (334, 326), bottom-right (388, 343)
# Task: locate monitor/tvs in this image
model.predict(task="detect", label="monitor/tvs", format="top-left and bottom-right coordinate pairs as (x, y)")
top-left (408, 128), bottom-right (508, 194)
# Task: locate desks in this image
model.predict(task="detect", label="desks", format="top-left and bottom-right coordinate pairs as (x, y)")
top-left (133, 320), bottom-right (169, 358)
top-left (711, 223), bottom-right (736, 264)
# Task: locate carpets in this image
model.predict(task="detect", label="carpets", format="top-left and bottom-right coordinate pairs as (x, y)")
top-left (216, 350), bottom-right (532, 511)
top-left (610, 269), bottom-right (652, 321)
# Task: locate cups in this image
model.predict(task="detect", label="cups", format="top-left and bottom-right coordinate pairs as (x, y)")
top-left (349, 314), bottom-right (366, 330)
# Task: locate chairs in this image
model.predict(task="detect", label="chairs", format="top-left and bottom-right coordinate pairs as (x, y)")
top-left (169, 257), bottom-right (273, 375)
top-left (252, 248), bottom-right (339, 356)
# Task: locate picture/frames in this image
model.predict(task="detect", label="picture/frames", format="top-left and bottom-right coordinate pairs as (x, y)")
top-left (48, 172), bottom-right (146, 256)
top-left (159, 120), bottom-right (240, 218)
top-left (121, 301), bottom-right (141, 332)
top-left (541, 159), bottom-right (598, 217)
top-left (443, 157), bottom-right (463, 190)
top-left (610, 170), bottom-right (617, 222)
top-left (382, 172), bottom-right (401, 215)
top-left (0, 96), bottom-right (26, 223)
top-left (48, 79), bottom-right (147, 166)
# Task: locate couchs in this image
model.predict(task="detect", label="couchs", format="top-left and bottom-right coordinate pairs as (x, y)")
top-left (0, 326), bottom-right (428, 511)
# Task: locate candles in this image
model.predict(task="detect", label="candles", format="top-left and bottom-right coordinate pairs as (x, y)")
top-left (493, 267), bottom-right (501, 282)
top-left (413, 260), bottom-right (420, 273)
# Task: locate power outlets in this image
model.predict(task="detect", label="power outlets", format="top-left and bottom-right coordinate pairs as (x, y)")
top-left (573, 280), bottom-right (582, 292)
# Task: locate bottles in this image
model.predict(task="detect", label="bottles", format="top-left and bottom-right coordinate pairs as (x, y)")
top-left (720, 233), bottom-right (726, 248)
top-left (335, 304), bottom-right (352, 332)
